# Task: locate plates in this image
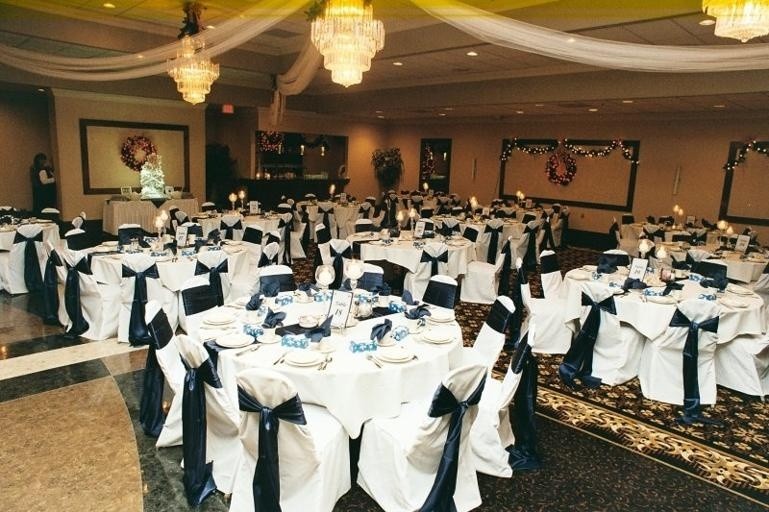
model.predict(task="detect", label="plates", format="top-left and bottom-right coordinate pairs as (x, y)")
top-left (750, 258), bottom-right (766, 263)
top-left (201, 290), bottom-right (456, 371)
top-left (356, 229), bottom-right (469, 247)
top-left (570, 264), bottom-right (753, 308)
top-left (93, 238), bottom-right (242, 265)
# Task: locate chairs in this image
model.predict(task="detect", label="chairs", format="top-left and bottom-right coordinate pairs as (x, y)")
top-left (231, 371), bottom-right (352, 509)
top-left (143, 300), bottom-right (192, 449)
top-left (171, 333), bottom-right (238, 497)
top-left (1, 191), bottom-right (769, 405)
top-left (463, 297), bottom-right (566, 479)
top-left (356, 362), bottom-right (488, 511)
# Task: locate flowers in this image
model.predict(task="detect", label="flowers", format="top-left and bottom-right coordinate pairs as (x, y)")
top-left (120, 136), bottom-right (156, 171)
top-left (547, 147), bottom-right (576, 183)
top-left (254, 130), bottom-right (285, 153)
top-left (422, 147), bottom-right (435, 181)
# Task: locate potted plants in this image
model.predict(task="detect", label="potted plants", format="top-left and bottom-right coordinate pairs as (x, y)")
top-left (370, 145), bottom-right (402, 186)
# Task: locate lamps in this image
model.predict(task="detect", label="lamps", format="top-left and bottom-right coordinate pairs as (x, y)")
top-left (310, 0), bottom-right (385, 88)
top-left (702, 0), bottom-right (769, 43)
top-left (166, 3), bottom-right (220, 106)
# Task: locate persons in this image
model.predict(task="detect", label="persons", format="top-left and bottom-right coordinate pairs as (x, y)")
top-left (29, 154), bottom-right (56, 217)
